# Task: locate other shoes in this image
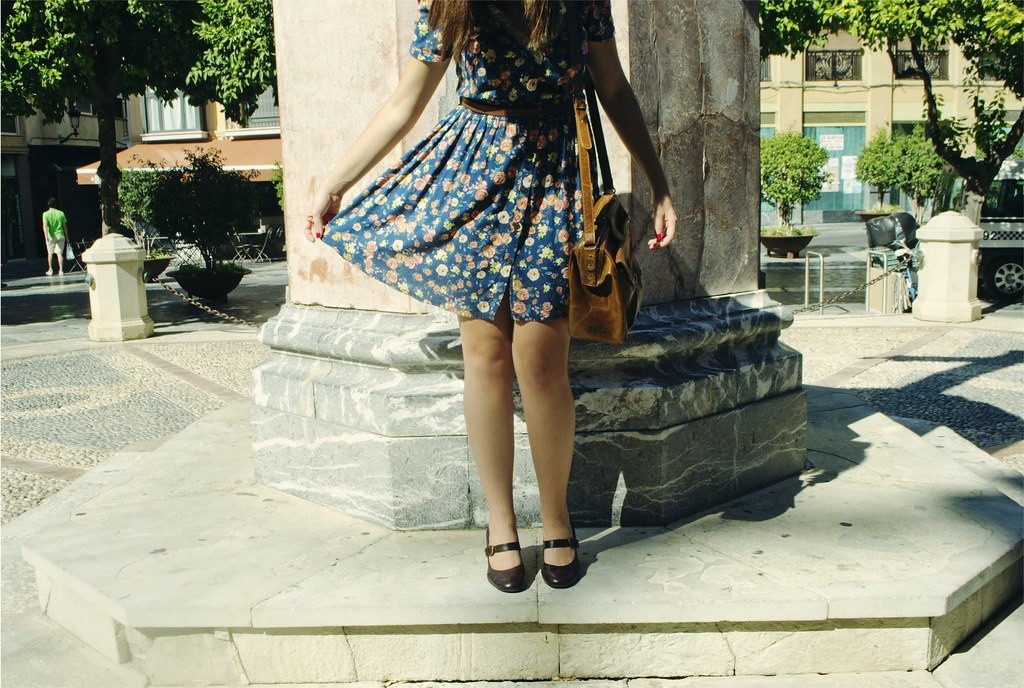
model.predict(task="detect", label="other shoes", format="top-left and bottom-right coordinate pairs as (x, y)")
top-left (45, 269), bottom-right (53, 276)
top-left (59, 271), bottom-right (64, 276)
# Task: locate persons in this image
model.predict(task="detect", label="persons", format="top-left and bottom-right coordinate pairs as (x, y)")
top-left (302, 0), bottom-right (678, 593)
top-left (41, 197), bottom-right (70, 276)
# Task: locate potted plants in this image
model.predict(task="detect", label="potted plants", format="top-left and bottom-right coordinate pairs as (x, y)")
top-left (853, 122), bottom-right (900, 220)
top-left (117, 146), bottom-right (264, 305)
top-left (759, 128), bottom-right (833, 258)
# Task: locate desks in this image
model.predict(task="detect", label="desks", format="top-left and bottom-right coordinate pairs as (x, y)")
top-left (147, 236), bottom-right (169, 257)
top-left (239, 231), bottom-right (266, 261)
top-left (76, 242), bottom-right (96, 272)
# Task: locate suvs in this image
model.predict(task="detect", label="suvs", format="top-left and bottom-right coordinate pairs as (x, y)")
top-left (919, 158), bottom-right (1024, 306)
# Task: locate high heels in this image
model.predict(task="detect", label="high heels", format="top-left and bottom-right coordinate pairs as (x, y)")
top-left (485, 527), bottom-right (525, 592)
top-left (542, 521), bottom-right (579, 588)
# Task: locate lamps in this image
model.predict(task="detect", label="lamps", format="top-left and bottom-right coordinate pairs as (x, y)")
top-left (58, 105), bottom-right (81, 145)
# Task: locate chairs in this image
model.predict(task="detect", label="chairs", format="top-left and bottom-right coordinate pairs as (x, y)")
top-left (225, 223), bottom-right (283, 264)
top-left (68, 237), bottom-right (95, 274)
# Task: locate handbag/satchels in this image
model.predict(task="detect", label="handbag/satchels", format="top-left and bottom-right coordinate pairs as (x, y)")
top-left (569, 192), bottom-right (643, 345)
top-left (66, 244), bottom-right (75, 261)
